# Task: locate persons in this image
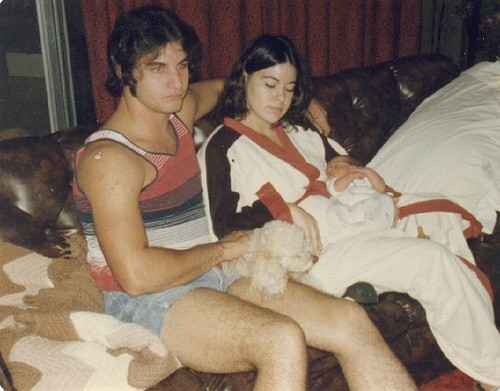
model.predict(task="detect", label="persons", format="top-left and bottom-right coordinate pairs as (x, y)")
top-left (199, 35), bottom-right (500, 391)
top-left (325, 155), bottom-right (394, 232)
top-left (72, 6), bottom-right (419, 391)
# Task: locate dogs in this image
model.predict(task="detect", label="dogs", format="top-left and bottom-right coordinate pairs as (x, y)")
top-left (0, 124), bottom-right (96, 259)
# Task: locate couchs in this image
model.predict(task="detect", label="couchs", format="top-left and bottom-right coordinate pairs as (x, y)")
top-left (0, 54), bottom-right (500, 391)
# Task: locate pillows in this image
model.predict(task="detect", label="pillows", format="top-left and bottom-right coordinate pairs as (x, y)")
top-left (366, 59), bottom-right (500, 236)
